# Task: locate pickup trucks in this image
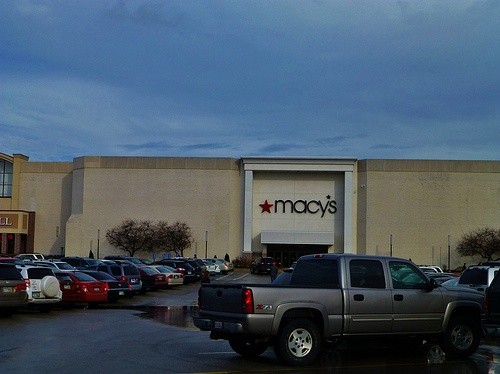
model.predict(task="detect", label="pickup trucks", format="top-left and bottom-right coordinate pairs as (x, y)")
top-left (191, 251), bottom-right (486, 369)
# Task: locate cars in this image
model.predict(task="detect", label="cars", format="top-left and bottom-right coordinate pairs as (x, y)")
top-left (350, 261), bottom-right (500, 328)
top-left (248, 257), bottom-right (276, 275)
top-left (0, 252), bottom-right (235, 315)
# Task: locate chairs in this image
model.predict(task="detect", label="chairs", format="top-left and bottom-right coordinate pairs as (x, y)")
top-left (350, 270), bottom-right (362, 287)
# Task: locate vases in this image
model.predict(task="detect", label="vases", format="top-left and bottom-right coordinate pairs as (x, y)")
top-left (451, 271), bottom-right (461, 277)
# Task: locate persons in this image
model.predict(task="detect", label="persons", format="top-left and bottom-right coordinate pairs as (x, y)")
top-left (270, 262), bottom-right (278, 284)
top-left (200, 268), bottom-right (210, 286)
